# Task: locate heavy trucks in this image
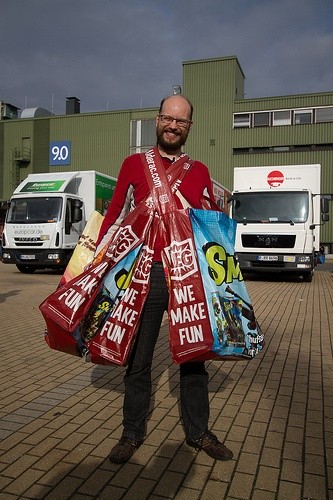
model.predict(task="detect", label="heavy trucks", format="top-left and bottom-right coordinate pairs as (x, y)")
top-left (216, 163), bottom-right (329, 282)
top-left (0, 169), bottom-right (118, 274)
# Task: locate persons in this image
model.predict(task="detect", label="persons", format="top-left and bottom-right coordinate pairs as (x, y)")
top-left (83, 93), bottom-right (235, 465)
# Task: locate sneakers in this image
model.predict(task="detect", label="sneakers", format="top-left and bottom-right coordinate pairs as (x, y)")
top-left (108, 428), bottom-right (146, 464)
top-left (187, 430), bottom-right (233, 461)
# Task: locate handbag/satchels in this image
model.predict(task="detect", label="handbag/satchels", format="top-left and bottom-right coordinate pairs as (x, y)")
top-left (38, 149), bottom-right (264, 365)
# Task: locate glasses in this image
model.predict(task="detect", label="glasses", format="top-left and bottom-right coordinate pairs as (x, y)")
top-left (157, 113), bottom-right (193, 128)
